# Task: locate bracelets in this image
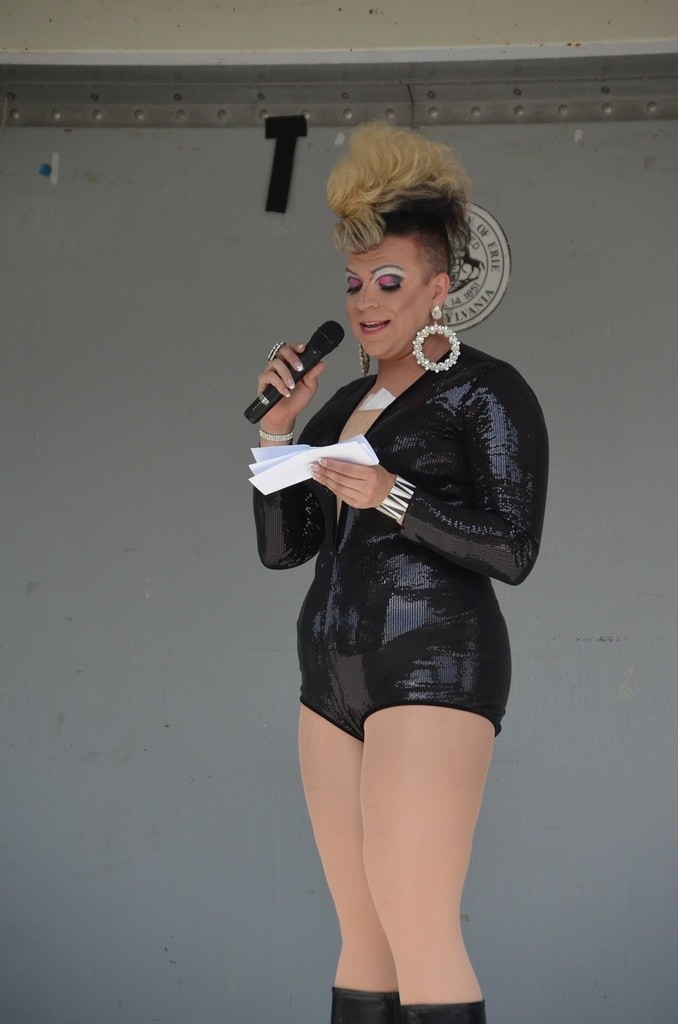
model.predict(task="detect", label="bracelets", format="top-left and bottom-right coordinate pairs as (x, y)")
top-left (260, 429), bottom-right (294, 441)
top-left (375, 475), bottom-right (417, 520)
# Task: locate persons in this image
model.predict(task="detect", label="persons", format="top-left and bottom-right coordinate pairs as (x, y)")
top-left (252, 122), bottom-right (549, 1024)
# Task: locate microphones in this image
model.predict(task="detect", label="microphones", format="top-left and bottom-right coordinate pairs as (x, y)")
top-left (243, 321), bottom-right (345, 424)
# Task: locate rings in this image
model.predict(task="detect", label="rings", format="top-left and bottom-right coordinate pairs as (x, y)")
top-left (268, 342), bottom-right (287, 366)
